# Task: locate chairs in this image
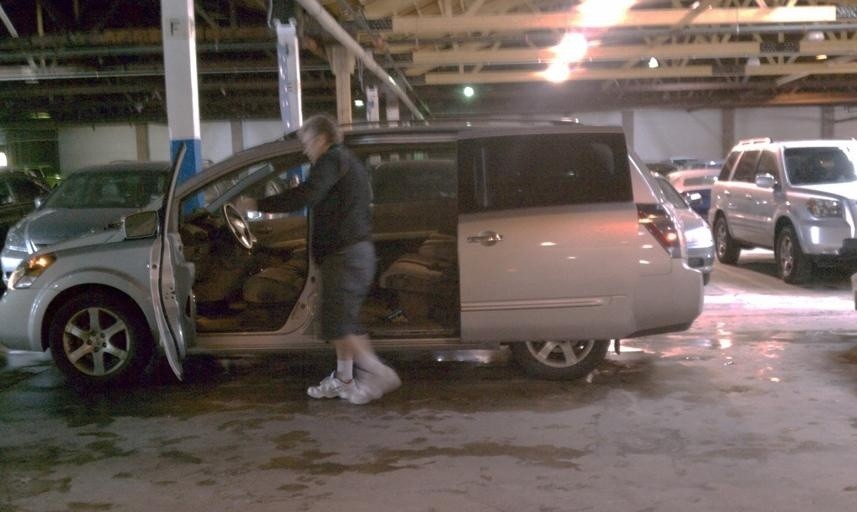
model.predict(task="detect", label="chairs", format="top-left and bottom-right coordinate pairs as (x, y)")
top-left (241, 246), bottom-right (309, 306)
top-left (96, 182), bottom-right (125, 207)
top-left (377, 229), bottom-right (457, 328)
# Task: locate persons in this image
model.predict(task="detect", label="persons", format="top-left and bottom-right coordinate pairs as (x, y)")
top-left (235, 113), bottom-right (403, 405)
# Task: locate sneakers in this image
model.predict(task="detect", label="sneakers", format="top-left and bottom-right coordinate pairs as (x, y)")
top-left (307, 365), bottom-right (402, 405)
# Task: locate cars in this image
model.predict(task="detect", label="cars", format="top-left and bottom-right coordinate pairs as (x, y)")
top-left (0, 160), bottom-right (218, 289)
top-left (641, 135), bottom-right (856, 285)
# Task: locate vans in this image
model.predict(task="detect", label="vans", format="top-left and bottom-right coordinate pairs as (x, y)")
top-left (0, 112), bottom-right (705, 383)
